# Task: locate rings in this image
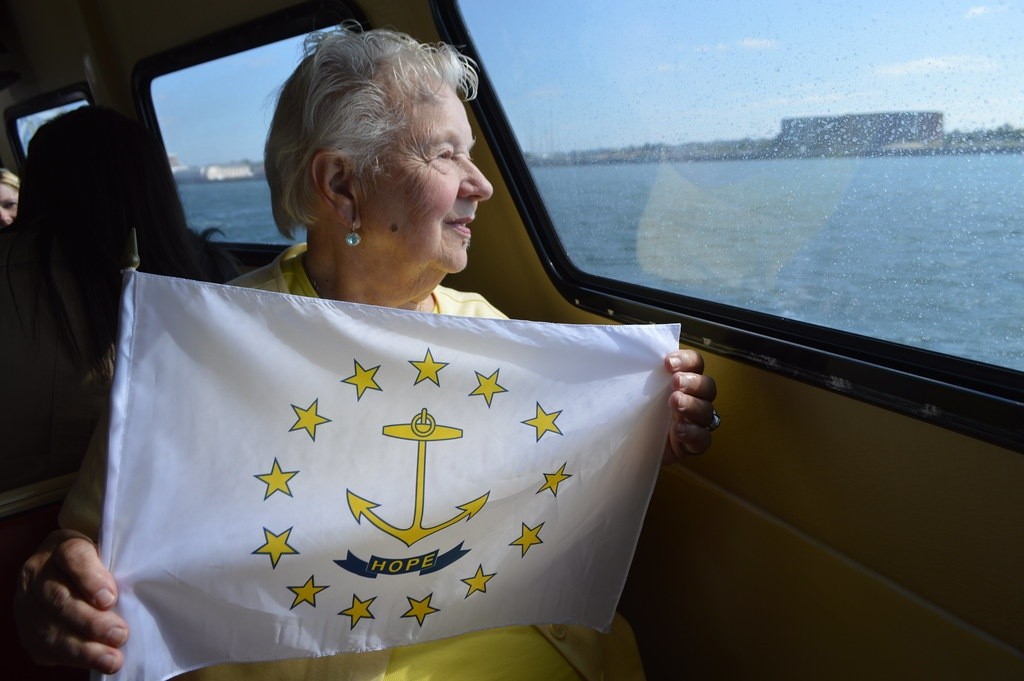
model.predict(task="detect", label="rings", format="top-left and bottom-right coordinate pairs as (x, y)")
top-left (706, 409), bottom-right (721, 431)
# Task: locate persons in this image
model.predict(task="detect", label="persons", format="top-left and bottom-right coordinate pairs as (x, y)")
top-left (0, 168), bottom-right (21, 228)
top-left (0, 110), bottom-right (241, 489)
top-left (16, 28), bottom-right (721, 680)
